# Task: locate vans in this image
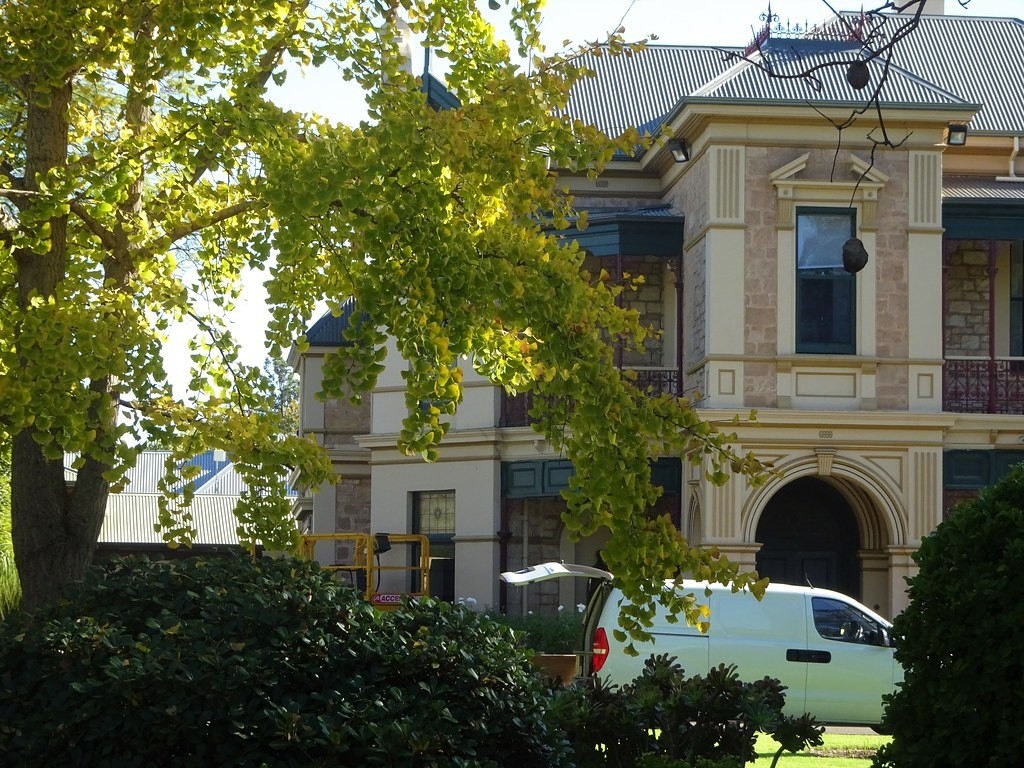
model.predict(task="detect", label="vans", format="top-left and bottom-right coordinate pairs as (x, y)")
top-left (500, 552), bottom-right (914, 735)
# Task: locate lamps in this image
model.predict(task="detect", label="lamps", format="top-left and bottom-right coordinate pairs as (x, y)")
top-left (945, 123), bottom-right (969, 147)
top-left (667, 136), bottom-right (691, 164)
